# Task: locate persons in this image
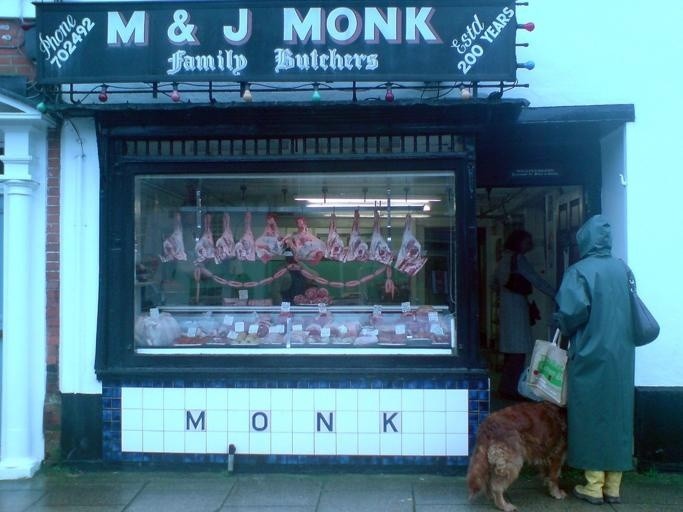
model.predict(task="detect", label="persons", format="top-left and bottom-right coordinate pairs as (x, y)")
top-left (496, 225), bottom-right (557, 407)
top-left (280, 239), bottom-right (314, 304)
top-left (553, 213), bottom-right (637, 504)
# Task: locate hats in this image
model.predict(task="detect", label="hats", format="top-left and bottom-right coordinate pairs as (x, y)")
top-left (505, 229), bottom-right (534, 250)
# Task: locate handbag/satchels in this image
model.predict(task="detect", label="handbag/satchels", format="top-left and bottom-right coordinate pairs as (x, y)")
top-left (624, 275), bottom-right (660, 347)
top-left (504, 269), bottom-right (533, 296)
top-left (517, 326), bottom-right (571, 409)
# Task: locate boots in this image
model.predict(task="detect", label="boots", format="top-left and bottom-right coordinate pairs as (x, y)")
top-left (602, 471), bottom-right (624, 504)
top-left (573, 468), bottom-right (605, 505)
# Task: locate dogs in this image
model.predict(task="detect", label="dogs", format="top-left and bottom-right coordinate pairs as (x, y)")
top-left (466, 400), bottom-right (568, 512)
